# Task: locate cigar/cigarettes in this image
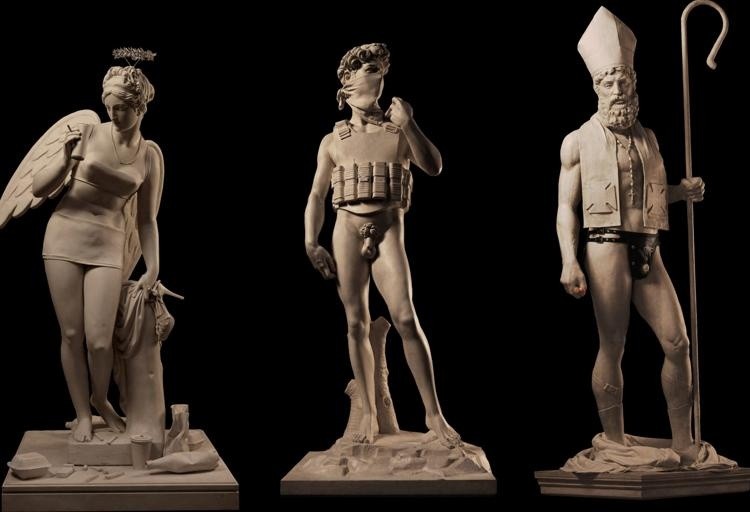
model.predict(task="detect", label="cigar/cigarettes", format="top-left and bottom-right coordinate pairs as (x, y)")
top-left (65, 123), bottom-right (73, 132)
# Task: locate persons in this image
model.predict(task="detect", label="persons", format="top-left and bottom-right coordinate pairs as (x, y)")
top-left (557, 8), bottom-right (737, 470)
top-left (32, 67), bottom-right (165, 443)
top-left (304, 40), bottom-right (465, 446)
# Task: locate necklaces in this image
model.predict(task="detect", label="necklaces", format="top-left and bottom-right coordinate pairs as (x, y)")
top-left (108, 122), bottom-right (143, 166)
top-left (613, 126), bottom-right (637, 205)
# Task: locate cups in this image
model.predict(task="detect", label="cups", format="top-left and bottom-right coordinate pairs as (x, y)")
top-left (131, 434), bottom-right (153, 469)
